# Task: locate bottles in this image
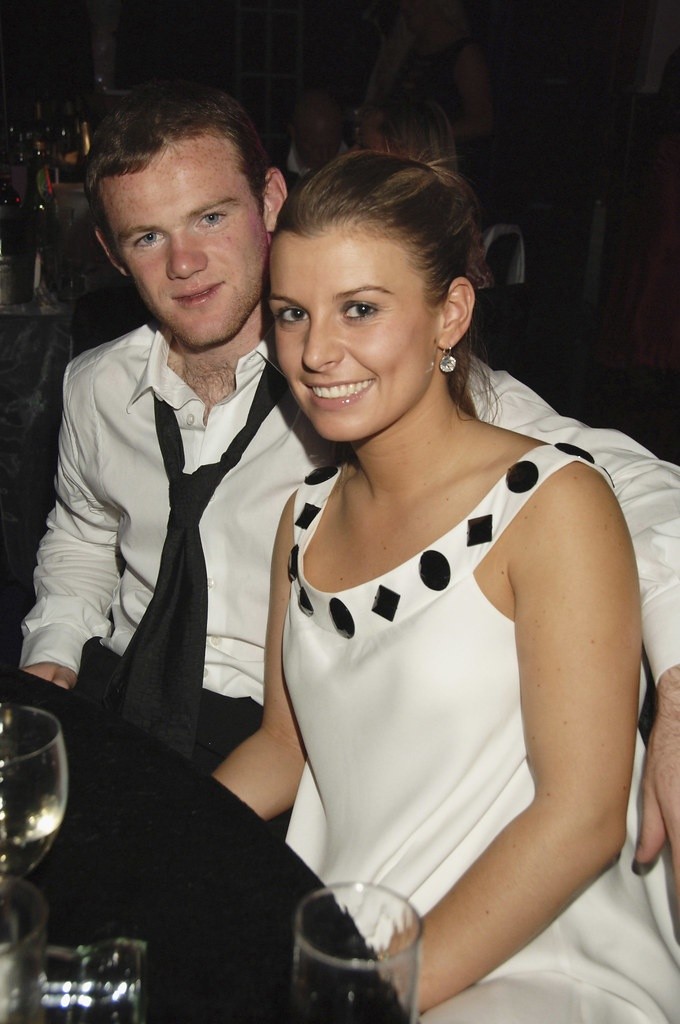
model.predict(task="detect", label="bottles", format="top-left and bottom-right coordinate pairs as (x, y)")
top-left (41, 937), bottom-right (150, 1024)
top-left (0, 98), bottom-right (93, 207)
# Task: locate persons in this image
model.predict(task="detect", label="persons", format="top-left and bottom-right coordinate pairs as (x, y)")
top-left (212, 145), bottom-right (680, 1024)
top-left (354, 0), bottom-right (492, 173)
top-left (17, 77), bottom-right (680, 914)
top-left (267, 90), bottom-right (343, 187)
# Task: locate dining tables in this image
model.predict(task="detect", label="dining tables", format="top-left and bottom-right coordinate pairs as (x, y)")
top-left (0, 660), bottom-right (415, 1024)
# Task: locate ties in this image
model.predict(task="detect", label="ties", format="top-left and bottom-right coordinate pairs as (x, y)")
top-left (100, 355), bottom-right (283, 757)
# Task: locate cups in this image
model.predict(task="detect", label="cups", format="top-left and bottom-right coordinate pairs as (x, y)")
top-left (0, 703), bottom-right (68, 885)
top-left (292, 883), bottom-right (422, 1024)
top-left (0, 876), bottom-right (49, 1024)
